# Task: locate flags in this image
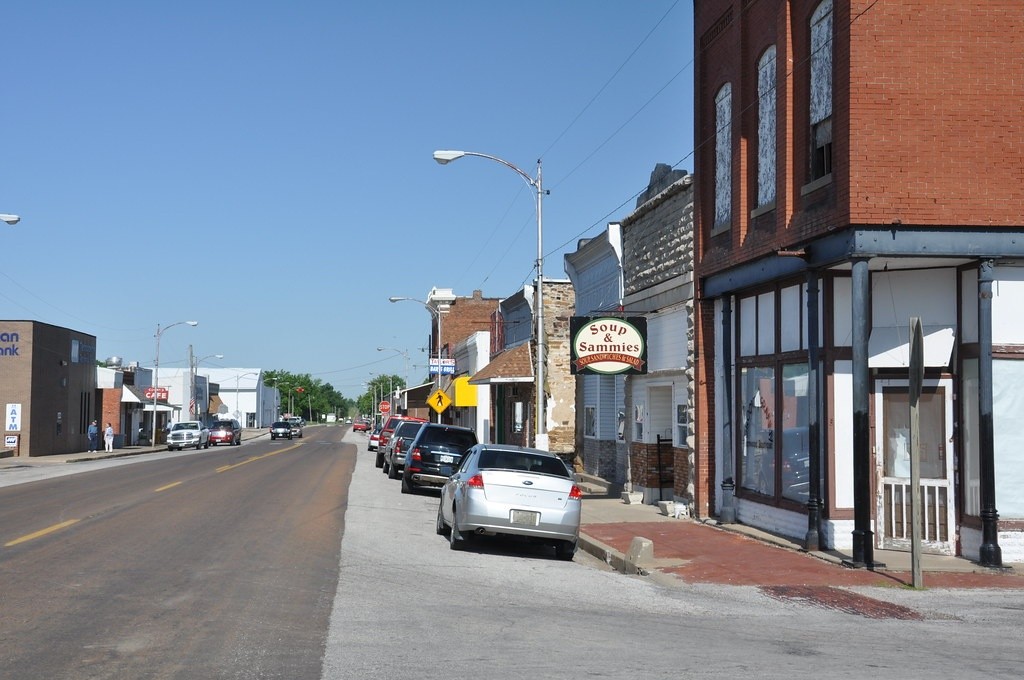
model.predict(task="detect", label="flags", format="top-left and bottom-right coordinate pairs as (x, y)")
top-left (190, 397), bottom-right (195, 414)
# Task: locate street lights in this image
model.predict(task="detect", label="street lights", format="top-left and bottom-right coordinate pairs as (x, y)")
top-left (236, 373), bottom-right (258, 420)
top-left (195, 354), bottom-right (224, 420)
top-left (259, 378), bottom-right (315, 429)
top-left (378, 347), bottom-right (409, 417)
top-left (433, 150), bottom-right (549, 455)
top-left (389, 297), bottom-right (442, 425)
top-left (362, 371), bottom-right (392, 430)
top-left (151, 320), bottom-right (198, 447)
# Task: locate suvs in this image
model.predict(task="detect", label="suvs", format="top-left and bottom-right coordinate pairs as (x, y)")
top-left (209, 419), bottom-right (242, 446)
top-left (167, 421), bottom-right (211, 451)
top-left (401, 421), bottom-right (479, 494)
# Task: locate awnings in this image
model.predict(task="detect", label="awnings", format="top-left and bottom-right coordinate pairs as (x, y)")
top-left (209, 393), bottom-right (228, 414)
top-left (121, 384), bottom-right (150, 404)
top-left (469, 344), bottom-right (534, 389)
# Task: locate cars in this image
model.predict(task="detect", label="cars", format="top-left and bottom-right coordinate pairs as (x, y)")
top-left (368, 414), bottom-right (430, 479)
top-left (339, 417), bottom-right (371, 433)
top-left (437, 444), bottom-right (581, 559)
top-left (269, 415), bottom-right (304, 440)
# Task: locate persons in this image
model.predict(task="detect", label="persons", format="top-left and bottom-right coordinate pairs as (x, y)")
top-left (166, 418), bottom-right (171, 432)
top-left (104, 423), bottom-right (114, 453)
top-left (87, 420), bottom-right (98, 453)
